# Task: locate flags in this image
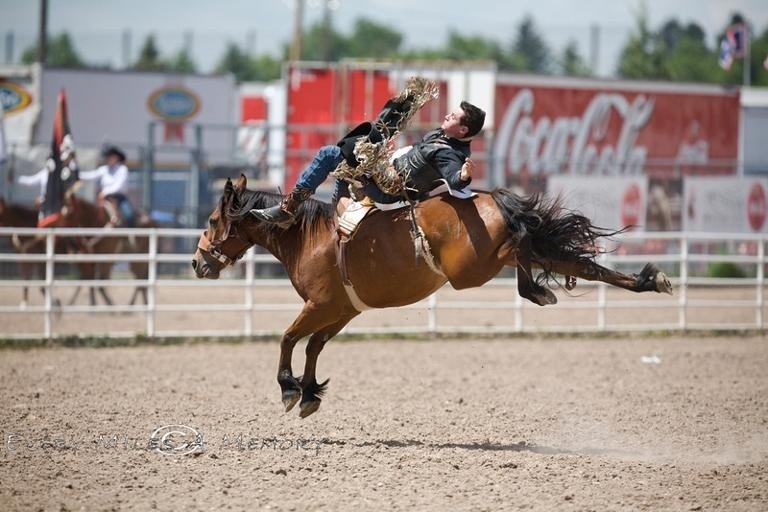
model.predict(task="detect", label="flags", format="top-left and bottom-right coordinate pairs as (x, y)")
top-left (36, 88), bottom-right (81, 227)
top-left (718, 22), bottom-right (748, 72)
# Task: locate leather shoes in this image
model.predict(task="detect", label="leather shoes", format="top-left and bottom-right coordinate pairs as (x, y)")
top-left (249, 202), bottom-right (296, 224)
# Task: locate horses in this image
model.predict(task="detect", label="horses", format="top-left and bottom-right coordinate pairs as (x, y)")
top-left (191, 172), bottom-right (675, 418)
top-left (1, 191), bottom-right (162, 305)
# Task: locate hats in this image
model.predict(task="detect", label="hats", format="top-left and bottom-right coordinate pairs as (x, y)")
top-left (103, 147), bottom-right (126, 161)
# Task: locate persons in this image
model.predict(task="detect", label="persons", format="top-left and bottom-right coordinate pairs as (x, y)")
top-left (77, 145), bottom-right (132, 226)
top-left (247, 74), bottom-right (490, 230)
top-left (16, 154), bottom-right (52, 195)
